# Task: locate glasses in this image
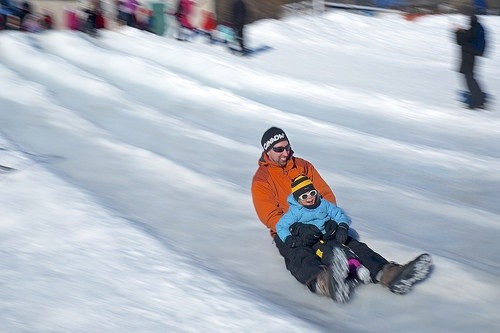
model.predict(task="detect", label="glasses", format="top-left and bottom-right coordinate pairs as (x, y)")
top-left (272, 144), bottom-right (291, 152)
top-left (299, 190), bottom-right (317, 199)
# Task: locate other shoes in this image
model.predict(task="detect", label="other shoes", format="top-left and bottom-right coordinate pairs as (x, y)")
top-left (358, 268), bottom-right (371, 284)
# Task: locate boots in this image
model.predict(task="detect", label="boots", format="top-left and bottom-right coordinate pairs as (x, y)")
top-left (380, 253), bottom-right (431, 294)
top-left (316, 247), bottom-right (352, 303)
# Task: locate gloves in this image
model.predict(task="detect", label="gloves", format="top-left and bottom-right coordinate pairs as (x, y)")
top-left (289, 223), bottom-right (324, 245)
top-left (323, 220), bottom-right (338, 240)
top-left (285, 235), bottom-right (306, 247)
top-left (336, 222), bottom-right (349, 244)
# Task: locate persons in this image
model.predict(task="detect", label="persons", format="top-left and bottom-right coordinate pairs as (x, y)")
top-left (454, 15), bottom-right (486, 109)
top-left (251, 127), bottom-right (434, 304)
top-left (275, 174), bottom-right (371, 286)
top-left (0, 0), bottom-right (246, 52)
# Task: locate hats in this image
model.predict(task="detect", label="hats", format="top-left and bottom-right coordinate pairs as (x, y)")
top-left (291, 174), bottom-right (314, 199)
top-left (261, 127), bottom-right (288, 151)
